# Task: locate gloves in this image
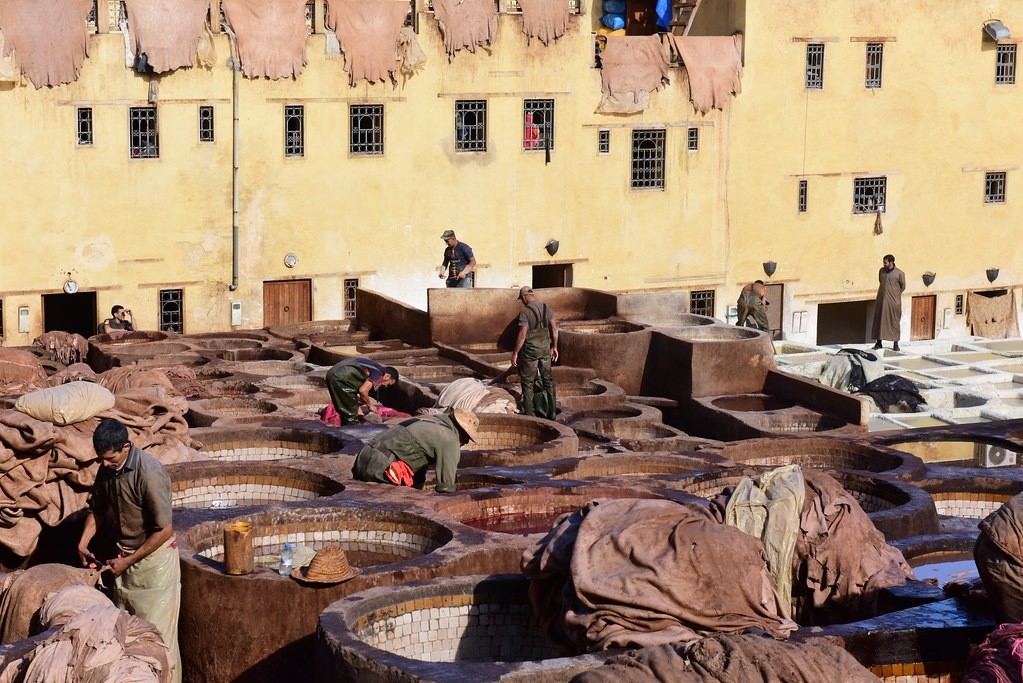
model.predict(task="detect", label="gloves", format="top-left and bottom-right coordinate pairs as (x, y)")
top-left (385, 460), bottom-right (414, 487)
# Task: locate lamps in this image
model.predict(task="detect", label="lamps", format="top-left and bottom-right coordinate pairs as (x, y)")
top-left (545, 239), bottom-right (559, 256)
top-left (987, 267), bottom-right (999, 282)
top-left (923, 271), bottom-right (936, 286)
top-left (137, 54), bottom-right (154, 72)
top-left (983, 19), bottom-right (1011, 40)
top-left (764, 261), bottom-right (777, 277)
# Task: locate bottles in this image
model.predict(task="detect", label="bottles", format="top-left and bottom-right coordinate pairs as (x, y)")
top-left (278, 544), bottom-right (293, 576)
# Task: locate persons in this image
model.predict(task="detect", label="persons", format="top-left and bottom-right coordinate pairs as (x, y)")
top-left (438, 230), bottom-right (476, 288)
top-left (356, 408), bottom-right (479, 493)
top-left (871, 255), bottom-right (906, 350)
top-left (103, 305), bottom-right (137, 334)
top-left (735, 280), bottom-right (771, 334)
top-left (511, 286), bottom-right (558, 419)
top-left (974, 491), bottom-right (1023, 624)
top-left (78, 418), bottom-right (183, 683)
top-left (326, 358), bottom-right (400, 426)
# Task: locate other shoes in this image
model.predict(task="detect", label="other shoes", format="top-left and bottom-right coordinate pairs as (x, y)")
top-left (872, 343), bottom-right (882, 349)
top-left (893, 342), bottom-right (900, 351)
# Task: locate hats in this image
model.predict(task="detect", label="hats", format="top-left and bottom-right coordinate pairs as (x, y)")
top-left (441, 229), bottom-right (454, 239)
top-left (450, 406), bottom-right (480, 443)
top-left (518, 286), bottom-right (535, 299)
top-left (291, 545), bottom-right (363, 583)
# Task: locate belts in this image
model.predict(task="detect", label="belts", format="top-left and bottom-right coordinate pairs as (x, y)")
top-left (367, 439), bottom-right (398, 463)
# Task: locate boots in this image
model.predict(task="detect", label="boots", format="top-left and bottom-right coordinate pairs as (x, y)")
top-left (524, 399), bottom-right (534, 416)
top-left (548, 395), bottom-right (556, 421)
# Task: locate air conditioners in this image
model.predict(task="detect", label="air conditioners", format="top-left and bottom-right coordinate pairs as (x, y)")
top-left (980, 442), bottom-right (1017, 469)
